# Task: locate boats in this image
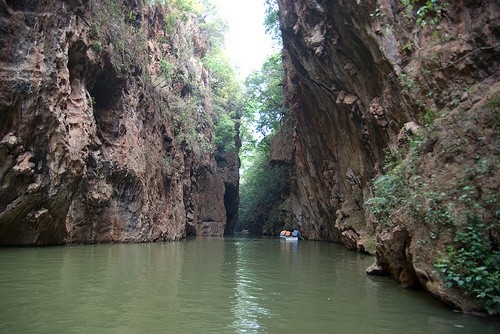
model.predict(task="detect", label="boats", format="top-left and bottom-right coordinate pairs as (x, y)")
top-left (280, 235), bottom-right (298, 241)
